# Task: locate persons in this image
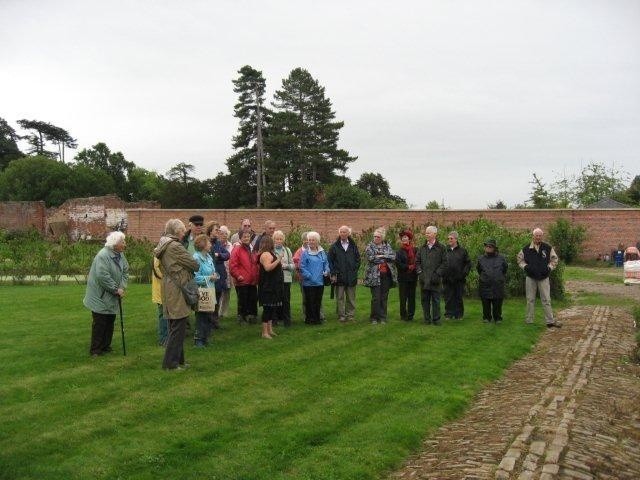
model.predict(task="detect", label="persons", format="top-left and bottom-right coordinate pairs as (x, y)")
top-left (255, 236), bottom-right (284, 339)
top-left (300, 231), bottom-right (330, 326)
top-left (205, 221), bottom-right (230, 330)
top-left (272, 231), bottom-right (295, 328)
top-left (229, 229), bottom-right (258, 324)
top-left (155, 219), bottom-right (200, 371)
top-left (218, 225), bottom-right (235, 317)
top-left (442, 231), bottom-right (471, 320)
top-left (83, 230), bottom-right (129, 355)
top-left (151, 249), bottom-right (169, 346)
top-left (364, 227), bottom-right (396, 325)
top-left (416, 226), bottom-right (450, 325)
top-left (193, 235), bottom-right (220, 345)
top-left (230, 219), bottom-right (255, 252)
top-left (477, 240), bottom-right (508, 324)
top-left (293, 232), bottom-right (308, 293)
top-left (252, 220), bottom-right (275, 252)
top-left (181, 215), bottom-right (204, 253)
top-left (326, 226), bottom-right (361, 322)
top-left (394, 231), bottom-right (421, 320)
top-left (516, 229), bottom-right (562, 328)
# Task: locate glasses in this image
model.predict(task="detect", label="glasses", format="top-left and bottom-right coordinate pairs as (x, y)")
top-left (243, 224), bottom-right (251, 228)
top-left (194, 223), bottom-right (203, 226)
top-left (374, 235), bottom-right (380, 239)
top-left (447, 237), bottom-right (453, 240)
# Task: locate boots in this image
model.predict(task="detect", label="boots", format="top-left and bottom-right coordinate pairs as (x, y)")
top-left (261, 321), bottom-right (273, 339)
top-left (265, 320), bottom-right (277, 338)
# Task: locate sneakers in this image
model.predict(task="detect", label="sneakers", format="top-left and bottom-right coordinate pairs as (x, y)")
top-left (347, 313), bottom-right (354, 321)
top-left (370, 318), bottom-right (378, 325)
top-left (381, 320), bottom-right (386, 325)
top-left (321, 318), bottom-right (328, 323)
top-left (339, 316), bottom-right (347, 323)
top-left (547, 320), bottom-right (562, 328)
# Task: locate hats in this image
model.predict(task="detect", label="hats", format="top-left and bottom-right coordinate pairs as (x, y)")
top-left (398, 230), bottom-right (413, 240)
top-left (189, 215), bottom-right (204, 225)
top-left (483, 239), bottom-right (499, 250)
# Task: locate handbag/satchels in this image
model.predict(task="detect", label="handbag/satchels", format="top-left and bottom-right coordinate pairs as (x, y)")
top-left (196, 276), bottom-right (217, 314)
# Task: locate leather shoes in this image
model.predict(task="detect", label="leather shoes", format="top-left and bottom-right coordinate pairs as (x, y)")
top-left (424, 320), bottom-right (432, 326)
top-left (163, 365), bottom-right (187, 371)
top-left (180, 360), bottom-right (192, 367)
top-left (456, 317), bottom-right (464, 322)
top-left (482, 319), bottom-right (489, 324)
top-left (89, 352), bottom-right (102, 358)
top-left (496, 319), bottom-right (504, 325)
top-left (445, 315), bottom-right (453, 321)
top-left (107, 349), bottom-right (118, 354)
top-left (433, 320), bottom-right (442, 327)
